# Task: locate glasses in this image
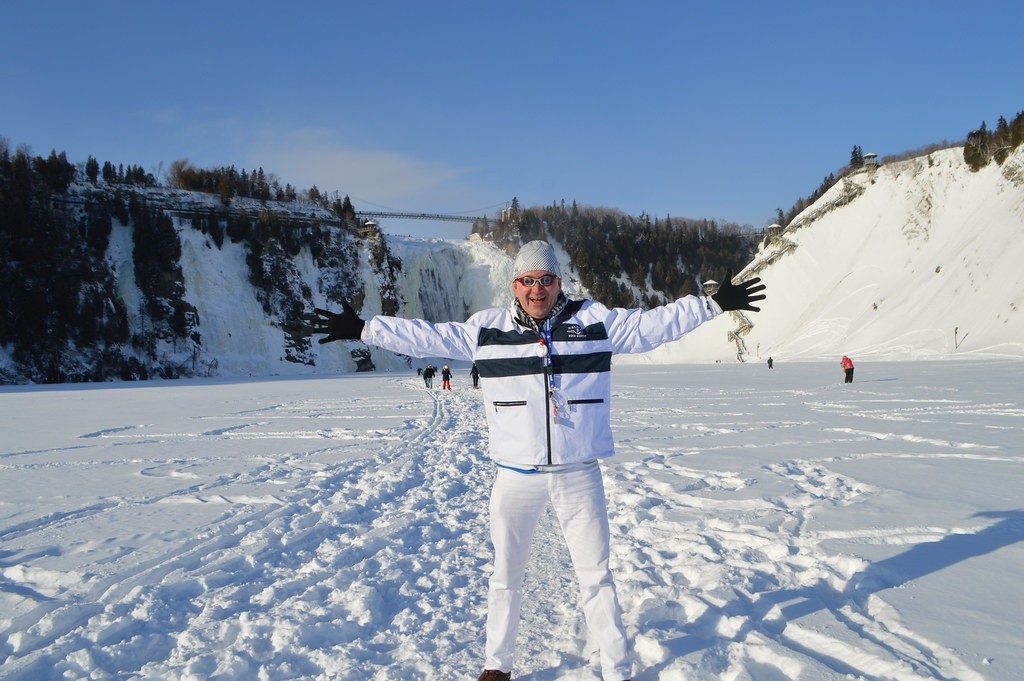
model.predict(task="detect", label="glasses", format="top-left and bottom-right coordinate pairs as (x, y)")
top-left (516, 274), bottom-right (559, 287)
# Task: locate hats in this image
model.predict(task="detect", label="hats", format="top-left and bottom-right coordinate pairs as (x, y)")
top-left (511, 240), bottom-right (563, 283)
top-left (444, 365), bottom-right (449, 371)
top-left (428, 365), bottom-right (433, 369)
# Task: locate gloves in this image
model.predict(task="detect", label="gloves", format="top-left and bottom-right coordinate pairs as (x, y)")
top-left (310, 297), bottom-right (365, 344)
top-left (711, 268), bottom-right (766, 312)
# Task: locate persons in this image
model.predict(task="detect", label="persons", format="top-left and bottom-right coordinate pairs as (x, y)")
top-left (768, 357), bottom-right (773, 369)
top-left (418, 365), bottom-right (438, 389)
top-left (309, 241), bottom-right (766, 681)
top-left (442, 365), bottom-right (452, 391)
top-left (470, 363), bottom-right (479, 389)
top-left (841, 356), bottom-right (854, 383)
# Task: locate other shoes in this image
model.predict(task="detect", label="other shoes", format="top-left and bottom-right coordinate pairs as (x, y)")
top-left (443, 388), bottom-right (445, 390)
top-left (449, 388), bottom-right (451, 391)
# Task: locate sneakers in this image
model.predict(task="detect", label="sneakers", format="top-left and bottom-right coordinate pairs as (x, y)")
top-left (478, 670), bottom-right (511, 681)
